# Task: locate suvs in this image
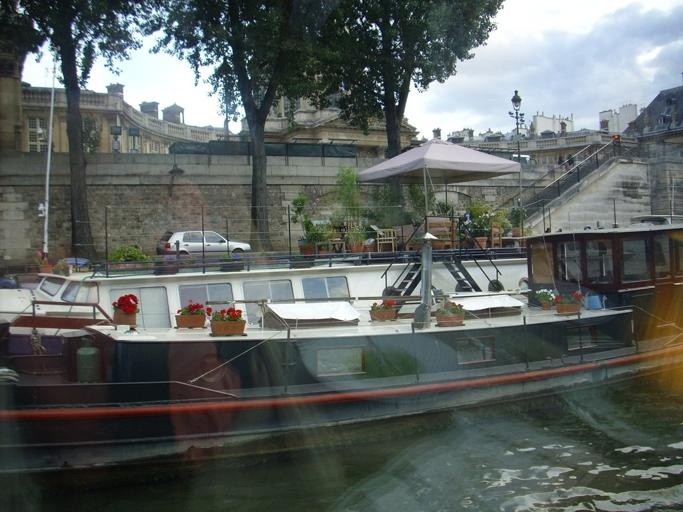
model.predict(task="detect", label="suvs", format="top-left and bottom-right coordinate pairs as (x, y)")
top-left (154, 227), bottom-right (250, 271)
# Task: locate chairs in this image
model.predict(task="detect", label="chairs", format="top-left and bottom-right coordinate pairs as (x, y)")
top-left (369, 225), bottom-right (399, 255)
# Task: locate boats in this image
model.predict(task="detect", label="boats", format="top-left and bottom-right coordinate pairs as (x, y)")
top-left (0, 214), bottom-right (683, 476)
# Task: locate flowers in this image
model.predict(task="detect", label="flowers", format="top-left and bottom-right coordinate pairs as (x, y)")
top-left (536, 288), bottom-right (586, 304)
top-left (112, 293), bottom-right (138, 314)
top-left (175, 302), bottom-right (244, 321)
top-left (434, 300), bottom-right (464, 314)
top-left (371, 297), bottom-right (397, 310)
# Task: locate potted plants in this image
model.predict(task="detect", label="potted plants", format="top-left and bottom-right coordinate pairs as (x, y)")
top-left (107, 245), bottom-right (155, 269)
top-left (289, 196), bottom-right (376, 259)
top-left (462, 199), bottom-right (527, 250)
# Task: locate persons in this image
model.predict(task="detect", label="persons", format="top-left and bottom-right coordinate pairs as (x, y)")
top-left (544, 225), bottom-right (550, 232)
top-left (0, 264), bottom-right (19, 289)
top-left (566, 151), bottom-right (573, 166)
top-left (555, 228), bottom-right (562, 232)
top-left (555, 155), bottom-right (572, 173)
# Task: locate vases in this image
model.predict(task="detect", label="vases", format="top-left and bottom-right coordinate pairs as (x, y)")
top-left (368, 309), bottom-right (399, 321)
top-left (174, 314), bottom-right (246, 335)
top-left (539, 300), bottom-right (581, 313)
top-left (435, 311), bottom-right (464, 327)
top-left (114, 307), bottom-right (136, 324)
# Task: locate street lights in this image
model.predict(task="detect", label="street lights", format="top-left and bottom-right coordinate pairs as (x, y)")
top-left (508, 88), bottom-right (524, 237)
top-left (215, 57), bottom-right (233, 143)
